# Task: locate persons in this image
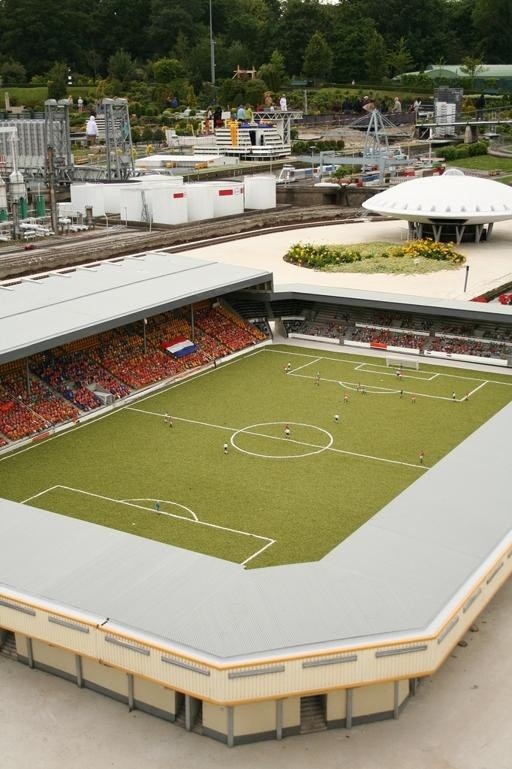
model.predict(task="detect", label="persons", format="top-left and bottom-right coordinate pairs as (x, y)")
top-left (340, 91), bottom-right (487, 123)
top-left (1, 292), bottom-right (512, 519)
top-left (206, 90), bottom-right (288, 128)
top-left (170, 97), bottom-right (178, 108)
top-left (66, 94), bottom-right (99, 146)
top-left (164, 96), bottom-right (172, 108)
